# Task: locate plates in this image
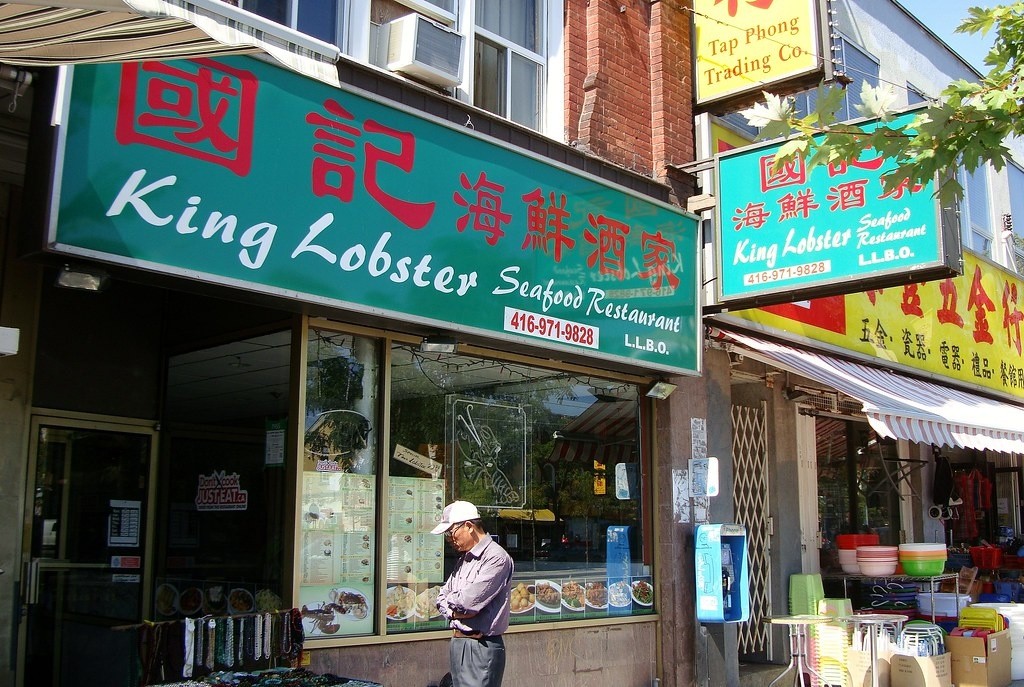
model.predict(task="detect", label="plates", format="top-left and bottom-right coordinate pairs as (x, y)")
top-left (228, 588), bottom-right (253, 614)
top-left (156, 584), bottom-right (178, 615)
top-left (415, 591), bottom-right (442, 618)
top-left (332, 587), bottom-right (370, 621)
top-left (561, 582), bottom-right (585, 612)
top-left (204, 591), bottom-right (229, 616)
top-left (386, 586), bottom-right (417, 620)
top-left (254, 593), bottom-right (282, 613)
top-left (631, 581), bottom-right (653, 606)
top-left (301, 602), bottom-right (337, 635)
top-left (609, 583), bottom-right (631, 608)
top-left (510, 585), bottom-right (535, 614)
top-left (178, 588), bottom-right (205, 614)
top-left (585, 583), bottom-right (609, 609)
top-left (535, 580), bottom-right (561, 613)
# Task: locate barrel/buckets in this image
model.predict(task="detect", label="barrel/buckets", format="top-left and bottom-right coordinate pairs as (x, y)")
top-left (971, 603), bottom-right (1024, 680)
top-left (919, 592), bottom-right (970, 617)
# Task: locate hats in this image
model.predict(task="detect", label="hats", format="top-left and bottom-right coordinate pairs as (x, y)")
top-left (430, 500), bottom-right (480, 535)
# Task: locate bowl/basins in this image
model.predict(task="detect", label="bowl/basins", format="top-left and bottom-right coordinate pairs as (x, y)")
top-left (899, 543), bottom-right (947, 576)
top-left (837, 534), bottom-right (898, 577)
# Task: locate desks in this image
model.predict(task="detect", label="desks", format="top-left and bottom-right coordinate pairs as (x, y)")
top-left (825, 571), bottom-right (959, 624)
top-left (835, 614), bottom-right (909, 687)
top-left (760, 614), bottom-right (833, 687)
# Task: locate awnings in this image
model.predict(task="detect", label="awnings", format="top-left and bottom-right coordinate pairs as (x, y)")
top-left (705, 324), bottom-right (1024, 458)
top-left (0, 0), bottom-right (344, 90)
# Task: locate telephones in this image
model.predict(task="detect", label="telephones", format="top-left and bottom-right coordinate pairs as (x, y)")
top-left (721, 544), bottom-right (735, 601)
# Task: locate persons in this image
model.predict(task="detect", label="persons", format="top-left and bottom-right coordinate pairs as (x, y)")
top-left (428, 500), bottom-right (515, 687)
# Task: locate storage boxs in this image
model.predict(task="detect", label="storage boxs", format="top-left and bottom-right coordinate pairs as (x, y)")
top-left (845, 650), bottom-right (894, 687)
top-left (944, 628), bottom-right (1012, 687)
top-left (889, 651), bottom-right (952, 687)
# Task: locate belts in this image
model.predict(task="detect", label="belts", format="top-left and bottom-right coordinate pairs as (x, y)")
top-left (453, 629), bottom-right (483, 639)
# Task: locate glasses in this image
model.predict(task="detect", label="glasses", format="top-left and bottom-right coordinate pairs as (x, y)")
top-left (444, 520), bottom-right (475, 536)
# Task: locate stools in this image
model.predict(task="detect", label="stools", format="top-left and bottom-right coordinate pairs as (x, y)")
top-left (785, 572), bottom-right (1013, 687)
